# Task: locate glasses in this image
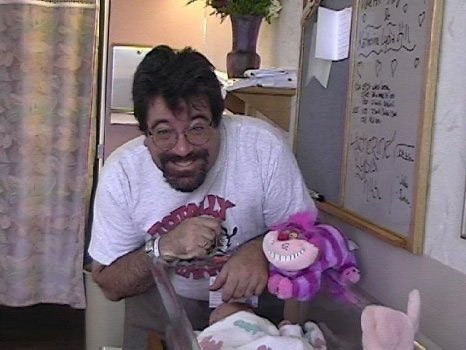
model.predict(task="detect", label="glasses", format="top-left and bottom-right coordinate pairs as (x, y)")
top-left (146, 119), bottom-right (214, 150)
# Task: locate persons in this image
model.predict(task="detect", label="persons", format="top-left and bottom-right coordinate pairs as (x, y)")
top-left (88, 44), bottom-right (319, 350)
top-left (196, 301), bottom-right (327, 350)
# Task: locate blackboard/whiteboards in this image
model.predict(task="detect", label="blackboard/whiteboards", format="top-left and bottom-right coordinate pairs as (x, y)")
top-left (292, 0), bottom-right (445, 256)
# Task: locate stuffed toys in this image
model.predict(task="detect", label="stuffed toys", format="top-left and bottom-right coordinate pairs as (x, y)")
top-left (260, 206), bottom-right (362, 302)
top-left (361, 289), bottom-right (421, 350)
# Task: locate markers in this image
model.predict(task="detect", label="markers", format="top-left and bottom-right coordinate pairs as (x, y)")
top-left (306, 187), bottom-right (325, 202)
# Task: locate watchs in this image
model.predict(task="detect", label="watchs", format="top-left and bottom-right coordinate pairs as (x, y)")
top-left (144, 237), bottom-right (157, 256)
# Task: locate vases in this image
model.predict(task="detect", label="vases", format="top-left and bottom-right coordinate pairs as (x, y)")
top-left (226, 12), bottom-right (262, 78)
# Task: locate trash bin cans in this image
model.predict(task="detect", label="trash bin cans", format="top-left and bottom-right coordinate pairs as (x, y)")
top-left (82, 263), bottom-right (125, 350)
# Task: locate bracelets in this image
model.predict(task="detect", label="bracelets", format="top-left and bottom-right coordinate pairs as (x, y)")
top-left (153, 235), bottom-right (162, 257)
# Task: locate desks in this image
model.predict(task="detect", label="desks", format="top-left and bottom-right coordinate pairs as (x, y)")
top-left (150, 255), bottom-right (440, 350)
